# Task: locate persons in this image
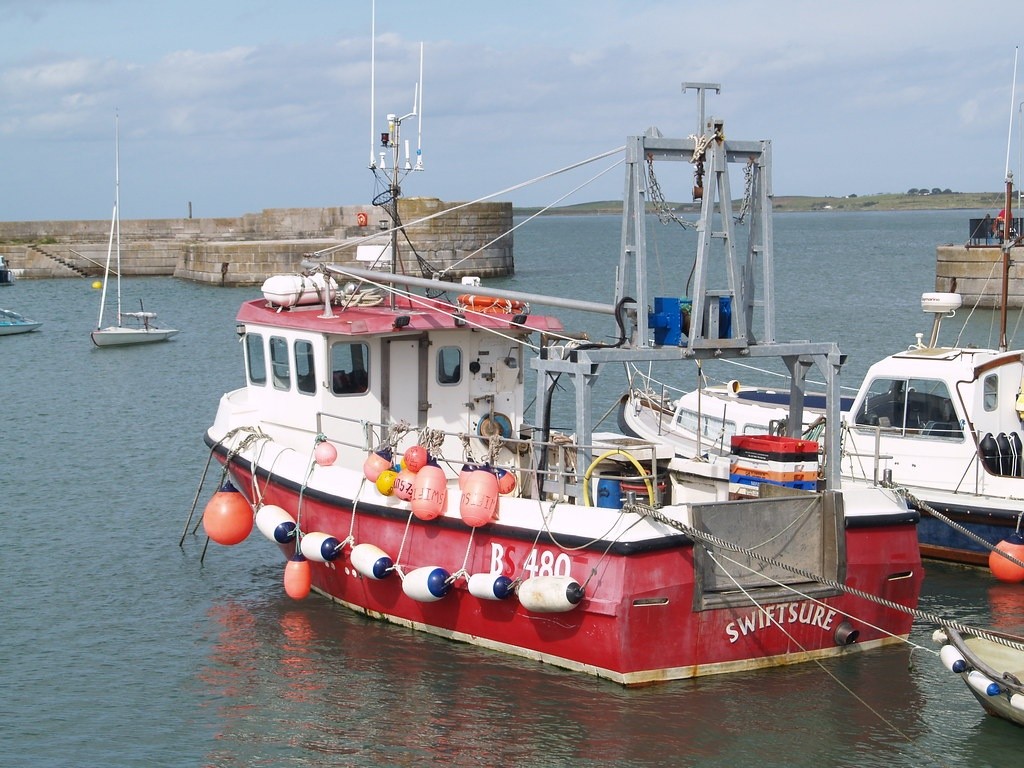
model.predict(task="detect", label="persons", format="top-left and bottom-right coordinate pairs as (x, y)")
top-left (983, 215), bottom-right (992, 245)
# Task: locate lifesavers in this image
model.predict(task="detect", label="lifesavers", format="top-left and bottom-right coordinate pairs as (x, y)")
top-left (357, 212), bottom-right (368, 226)
top-left (457, 294), bottom-right (528, 309)
top-left (459, 305), bottom-right (523, 316)
top-left (992, 218), bottom-right (1005, 237)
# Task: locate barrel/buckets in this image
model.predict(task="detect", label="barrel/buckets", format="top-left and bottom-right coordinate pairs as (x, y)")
top-left (597, 465), bottom-right (667, 509)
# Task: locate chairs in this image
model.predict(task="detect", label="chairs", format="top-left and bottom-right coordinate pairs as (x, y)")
top-left (332, 369), bottom-right (368, 394)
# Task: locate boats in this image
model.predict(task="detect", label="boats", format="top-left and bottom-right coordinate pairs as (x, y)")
top-left (611, 41), bottom-right (1024, 567)
top-left (932, 622), bottom-right (1024, 731)
top-left (0, 305), bottom-right (43, 337)
top-left (202, 0), bottom-right (927, 690)
top-left (0, 255), bottom-right (17, 286)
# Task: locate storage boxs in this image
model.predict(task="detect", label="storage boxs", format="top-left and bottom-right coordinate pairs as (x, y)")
top-left (729, 434), bottom-right (819, 502)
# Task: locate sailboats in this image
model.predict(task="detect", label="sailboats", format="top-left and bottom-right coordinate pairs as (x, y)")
top-left (89, 108), bottom-right (180, 347)
